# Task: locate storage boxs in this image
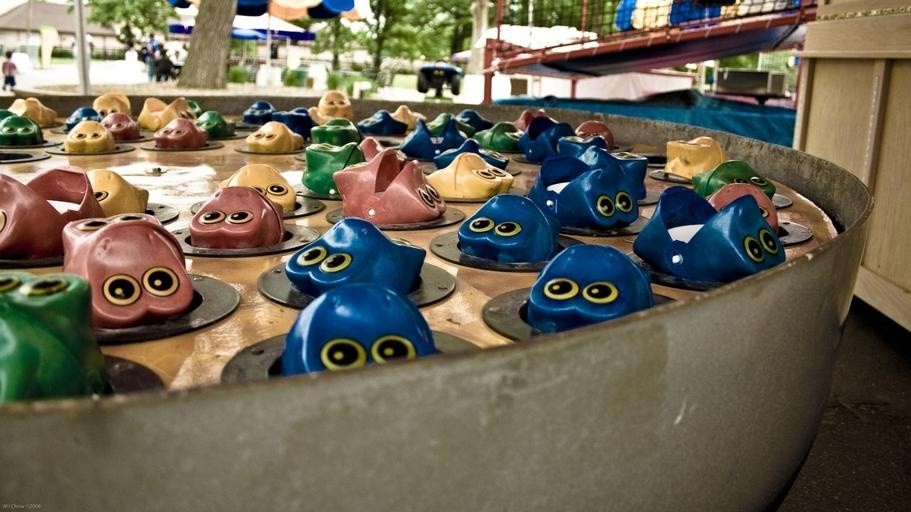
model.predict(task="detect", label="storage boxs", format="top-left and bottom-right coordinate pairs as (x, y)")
top-left (782, 0), bottom-right (911, 337)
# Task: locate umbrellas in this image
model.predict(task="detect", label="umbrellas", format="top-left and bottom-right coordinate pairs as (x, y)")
top-left (169, 1), bottom-right (373, 69)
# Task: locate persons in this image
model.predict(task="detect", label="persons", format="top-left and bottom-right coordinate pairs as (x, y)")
top-left (142, 34), bottom-right (178, 83)
top-left (2, 51), bottom-right (18, 91)
